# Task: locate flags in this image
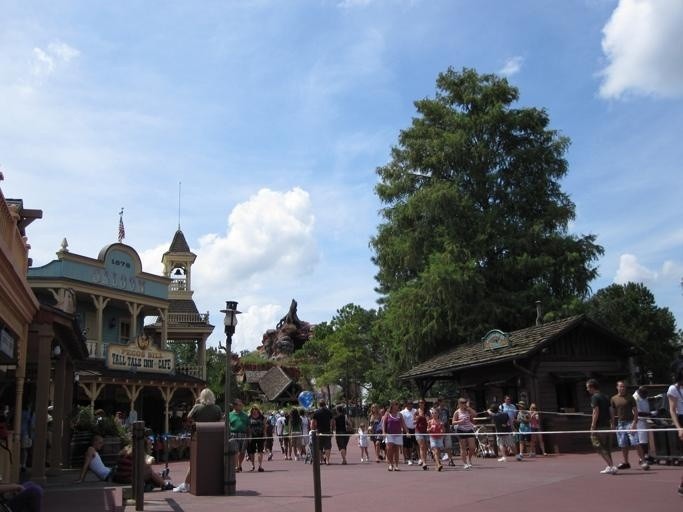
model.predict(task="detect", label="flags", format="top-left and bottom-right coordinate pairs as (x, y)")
top-left (118, 216), bottom-right (125, 241)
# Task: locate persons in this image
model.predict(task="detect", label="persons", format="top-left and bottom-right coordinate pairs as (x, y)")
top-left (585, 377), bottom-right (683, 494)
top-left (186, 388), bottom-right (355, 471)
top-left (358, 397), bottom-right (457, 471)
top-left (452, 393), bottom-right (549, 469)
top-left (0, 403), bottom-right (176, 511)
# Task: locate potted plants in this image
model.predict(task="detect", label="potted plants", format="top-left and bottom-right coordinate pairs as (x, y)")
top-left (96, 413), bottom-right (133, 469)
top-left (69, 404), bottom-right (95, 467)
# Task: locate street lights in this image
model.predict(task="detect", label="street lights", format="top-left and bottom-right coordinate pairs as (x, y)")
top-left (217, 300), bottom-right (241, 495)
top-left (338, 361), bottom-right (349, 431)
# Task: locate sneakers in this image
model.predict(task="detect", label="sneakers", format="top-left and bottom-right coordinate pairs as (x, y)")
top-left (161, 481), bottom-right (175, 489)
top-left (618, 463), bottom-right (630, 469)
top-left (361, 451), bottom-right (548, 472)
top-left (600, 467), bottom-right (616, 473)
top-left (235, 450), bottom-right (347, 472)
top-left (173, 484), bottom-right (189, 493)
top-left (641, 463), bottom-right (650, 469)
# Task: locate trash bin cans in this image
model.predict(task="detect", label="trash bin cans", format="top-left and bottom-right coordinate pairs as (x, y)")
top-left (190, 422), bottom-right (227, 495)
top-left (649, 426), bottom-right (681, 465)
top-left (450, 424), bottom-right (498, 457)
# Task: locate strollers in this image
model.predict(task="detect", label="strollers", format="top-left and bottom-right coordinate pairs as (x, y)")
top-left (304, 439), bottom-right (327, 466)
top-left (471, 424), bottom-right (495, 458)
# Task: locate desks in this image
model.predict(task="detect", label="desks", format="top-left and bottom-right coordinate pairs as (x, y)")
top-left (152, 434), bottom-right (191, 462)
